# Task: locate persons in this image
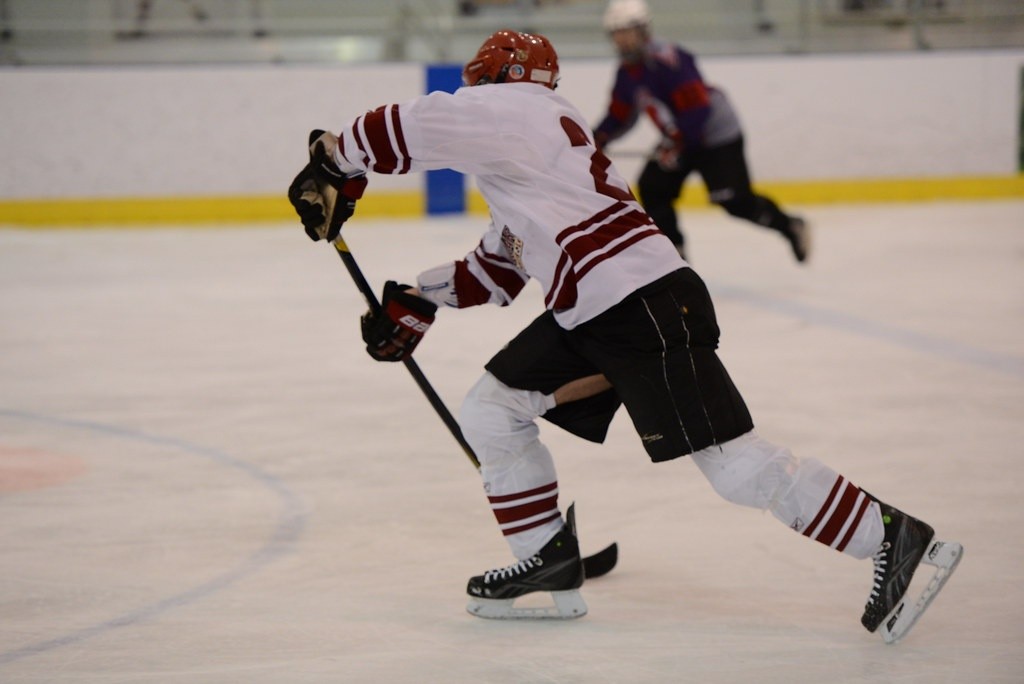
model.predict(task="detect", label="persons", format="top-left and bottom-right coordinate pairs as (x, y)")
top-left (592, 0), bottom-right (810, 261)
top-left (286, 30), bottom-right (964, 644)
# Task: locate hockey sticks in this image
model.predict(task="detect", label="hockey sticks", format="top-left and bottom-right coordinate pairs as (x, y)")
top-left (603, 147), bottom-right (664, 162)
top-left (298, 179), bottom-right (619, 579)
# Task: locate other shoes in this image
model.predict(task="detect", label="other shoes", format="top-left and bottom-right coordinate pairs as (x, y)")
top-left (788, 218), bottom-right (808, 260)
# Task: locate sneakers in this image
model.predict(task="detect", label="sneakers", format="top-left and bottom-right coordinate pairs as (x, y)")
top-left (859, 486), bottom-right (964, 644)
top-left (467, 502), bottom-right (586, 619)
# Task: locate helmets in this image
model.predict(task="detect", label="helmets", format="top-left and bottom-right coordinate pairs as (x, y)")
top-left (603, 0), bottom-right (648, 34)
top-left (462, 29), bottom-right (560, 91)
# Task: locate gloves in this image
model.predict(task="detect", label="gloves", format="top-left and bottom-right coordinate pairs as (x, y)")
top-left (361, 281), bottom-right (440, 363)
top-left (291, 129), bottom-right (367, 243)
